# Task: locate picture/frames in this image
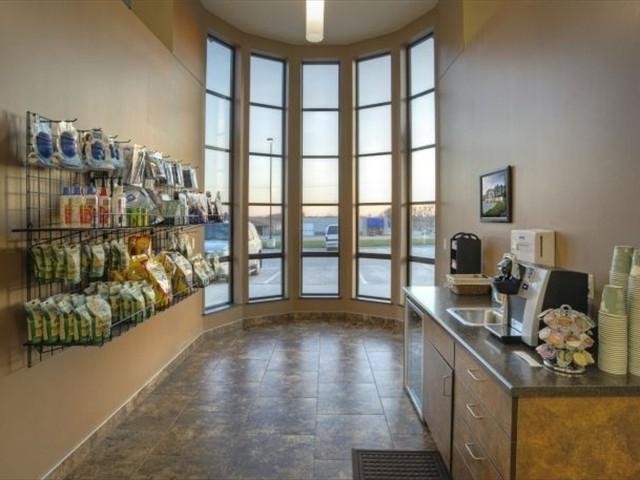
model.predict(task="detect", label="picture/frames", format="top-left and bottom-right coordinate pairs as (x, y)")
top-left (478, 164), bottom-right (513, 223)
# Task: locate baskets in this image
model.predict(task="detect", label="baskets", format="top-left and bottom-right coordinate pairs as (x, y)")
top-left (447, 273), bottom-right (490, 296)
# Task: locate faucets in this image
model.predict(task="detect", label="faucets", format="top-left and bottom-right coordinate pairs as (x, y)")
top-left (481, 271), bottom-right (504, 305)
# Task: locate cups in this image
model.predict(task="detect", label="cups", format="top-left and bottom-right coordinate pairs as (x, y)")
top-left (597, 244), bottom-right (640, 376)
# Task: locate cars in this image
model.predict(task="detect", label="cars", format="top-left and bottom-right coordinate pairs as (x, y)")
top-left (325, 224), bottom-right (339, 251)
top-left (203, 221), bottom-right (263, 283)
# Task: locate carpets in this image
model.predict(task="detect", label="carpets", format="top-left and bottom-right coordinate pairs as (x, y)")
top-left (351, 448), bottom-right (451, 480)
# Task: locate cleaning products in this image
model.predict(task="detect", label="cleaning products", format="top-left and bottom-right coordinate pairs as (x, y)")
top-left (60, 182), bottom-right (125, 228)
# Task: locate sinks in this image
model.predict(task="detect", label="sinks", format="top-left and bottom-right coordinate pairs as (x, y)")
top-left (446, 304), bottom-right (503, 327)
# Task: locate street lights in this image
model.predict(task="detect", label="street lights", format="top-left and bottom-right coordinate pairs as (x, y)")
top-left (265, 137), bottom-right (275, 248)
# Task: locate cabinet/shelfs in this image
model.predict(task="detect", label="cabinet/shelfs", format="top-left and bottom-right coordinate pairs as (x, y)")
top-left (12, 208), bottom-right (218, 346)
top-left (451, 340), bottom-right (640, 479)
top-left (403, 296), bottom-right (423, 424)
top-left (424, 310), bottom-right (455, 480)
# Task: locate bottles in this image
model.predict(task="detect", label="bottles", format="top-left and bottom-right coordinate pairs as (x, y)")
top-left (60, 182), bottom-right (126, 228)
